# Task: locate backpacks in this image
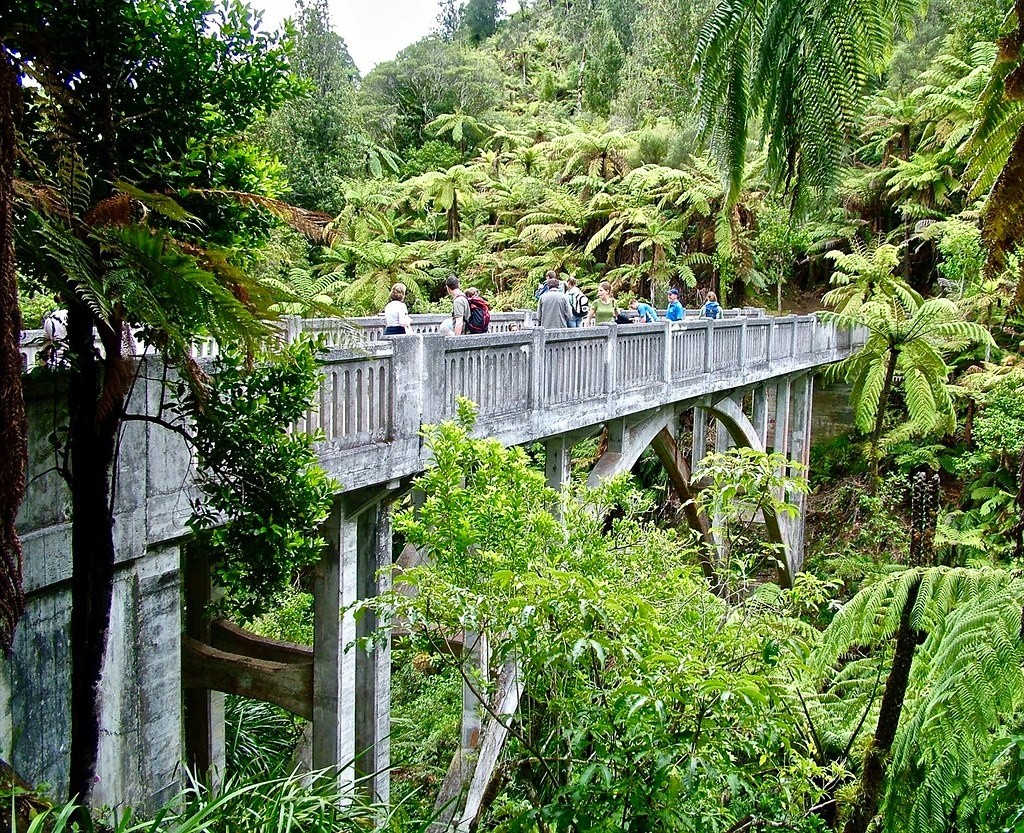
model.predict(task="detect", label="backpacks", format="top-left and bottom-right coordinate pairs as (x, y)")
top-left (705, 303), bottom-right (719, 319)
top-left (452, 292), bottom-right (490, 334)
top-left (570, 288), bottom-right (590, 318)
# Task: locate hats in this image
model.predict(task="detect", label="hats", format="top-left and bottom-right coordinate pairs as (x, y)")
top-left (667, 288), bottom-right (678, 294)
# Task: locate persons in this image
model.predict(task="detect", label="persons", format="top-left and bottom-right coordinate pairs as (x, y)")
top-left (665, 289), bottom-right (685, 322)
top-left (586, 281), bottom-right (627, 326)
top-left (382, 282), bottom-right (413, 335)
top-left (445, 274), bottom-right (492, 337)
top-left (628, 298), bottom-right (658, 324)
top-left (699, 291), bottom-right (723, 320)
top-left (42, 291), bottom-right (137, 368)
top-left (535, 271), bottom-right (589, 330)
